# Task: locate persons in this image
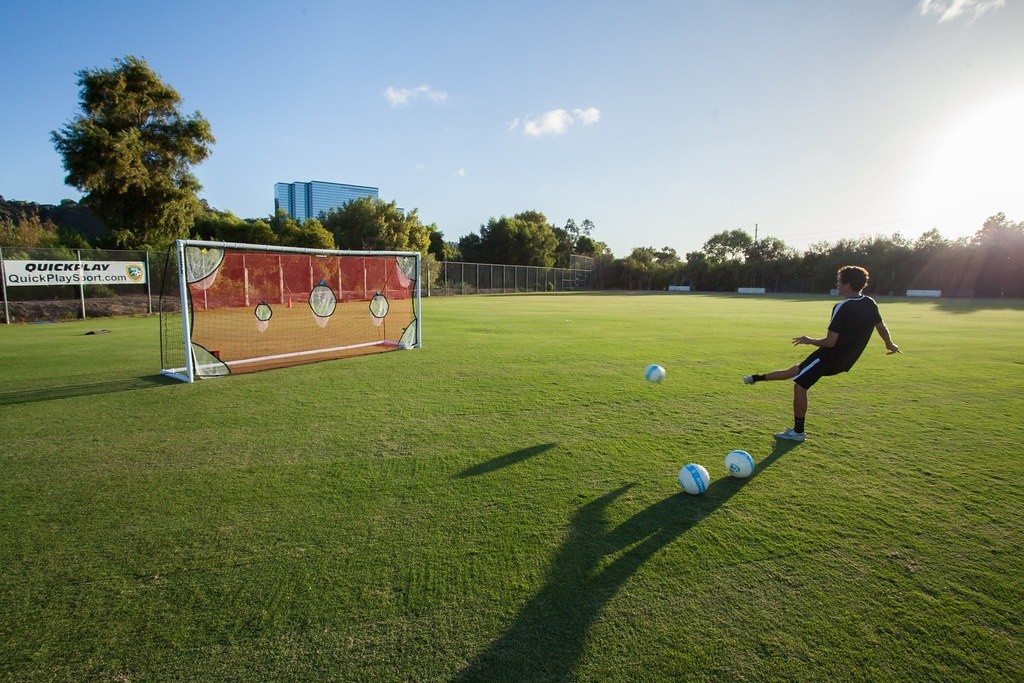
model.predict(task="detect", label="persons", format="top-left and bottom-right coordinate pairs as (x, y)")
top-left (743, 264), bottom-right (902, 442)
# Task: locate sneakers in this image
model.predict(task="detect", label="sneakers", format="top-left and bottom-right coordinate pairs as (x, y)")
top-left (743, 375), bottom-right (755, 385)
top-left (773, 427), bottom-right (807, 441)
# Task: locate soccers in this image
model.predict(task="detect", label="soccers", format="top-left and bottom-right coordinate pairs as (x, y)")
top-left (644, 364), bottom-right (666, 383)
top-left (724, 449), bottom-right (756, 479)
top-left (678, 463), bottom-right (711, 496)
